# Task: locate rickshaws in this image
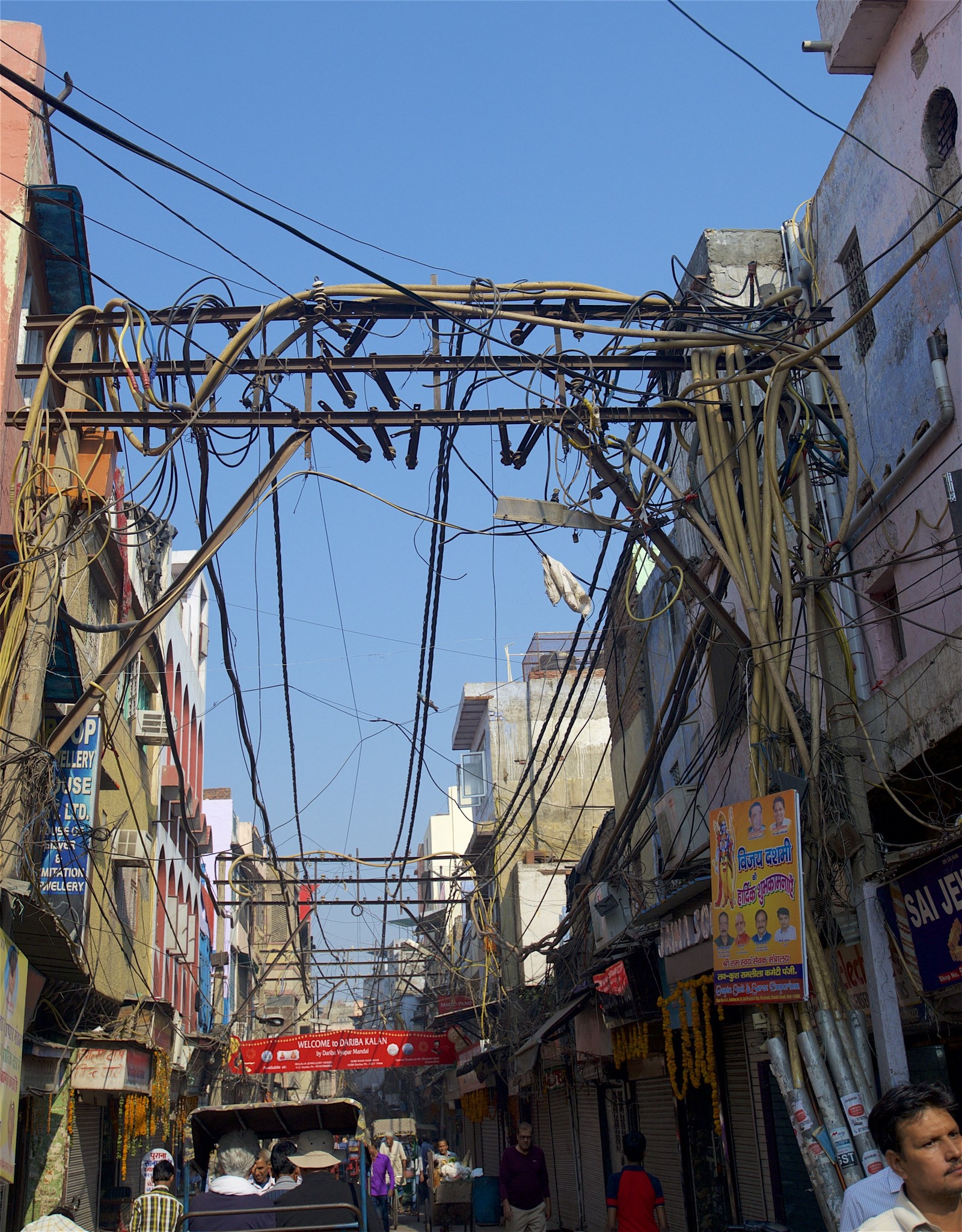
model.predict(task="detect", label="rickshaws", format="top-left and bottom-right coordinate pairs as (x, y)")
top-left (423, 1150), bottom-right (479, 1224)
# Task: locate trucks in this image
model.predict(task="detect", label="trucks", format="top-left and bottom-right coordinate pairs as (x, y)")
top-left (184, 1096), bottom-right (364, 1232)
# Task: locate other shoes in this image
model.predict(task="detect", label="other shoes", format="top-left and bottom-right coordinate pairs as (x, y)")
top-left (413, 1211), bottom-right (417, 1216)
top-left (399, 1205), bottom-right (404, 1213)
top-left (406, 1204), bottom-right (411, 1211)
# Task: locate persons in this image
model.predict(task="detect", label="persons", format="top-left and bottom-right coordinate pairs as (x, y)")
top-left (262, 1141), bottom-right (301, 1200)
top-left (426, 1138), bottom-right (459, 1232)
top-left (752, 909), bottom-right (772, 942)
top-left (367, 1140), bottom-right (395, 1232)
top-left (129, 1159), bottom-right (184, 1232)
top-left (273, 1130), bottom-right (385, 1232)
top-left (20, 1207), bottom-right (89, 1232)
top-left (748, 802), bottom-right (766, 833)
top-left (840, 1166), bottom-right (904, 1232)
top-left (333, 1134), bottom-right (359, 1178)
top-left (735, 912), bottom-right (750, 945)
top-left (775, 907), bottom-right (796, 940)
top-left (499, 1122), bottom-right (552, 1232)
top-left (852, 1083), bottom-right (962, 1232)
top-left (714, 813), bottom-right (735, 909)
top-left (604, 1130), bottom-right (670, 1232)
top-left (248, 1148), bottom-right (275, 1194)
top-left (770, 796), bottom-right (790, 830)
top-left (189, 1129), bottom-right (276, 1232)
top-left (714, 911), bottom-right (735, 947)
top-left (379, 1130), bottom-right (439, 1215)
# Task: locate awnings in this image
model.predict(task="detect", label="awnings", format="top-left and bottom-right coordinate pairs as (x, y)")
top-left (457, 1045), bottom-right (510, 1077)
top-left (424, 1073), bottom-right (445, 1102)
top-left (515, 991), bottom-right (608, 1058)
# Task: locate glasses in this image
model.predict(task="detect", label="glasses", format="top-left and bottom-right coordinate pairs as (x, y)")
top-left (517, 1131), bottom-right (533, 1140)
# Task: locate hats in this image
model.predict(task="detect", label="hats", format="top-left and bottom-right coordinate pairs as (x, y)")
top-left (286, 1130), bottom-right (346, 1168)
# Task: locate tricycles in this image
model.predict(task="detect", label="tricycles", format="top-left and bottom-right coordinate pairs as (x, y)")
top-left (371, 1118), bottom-right (420, 1231)
